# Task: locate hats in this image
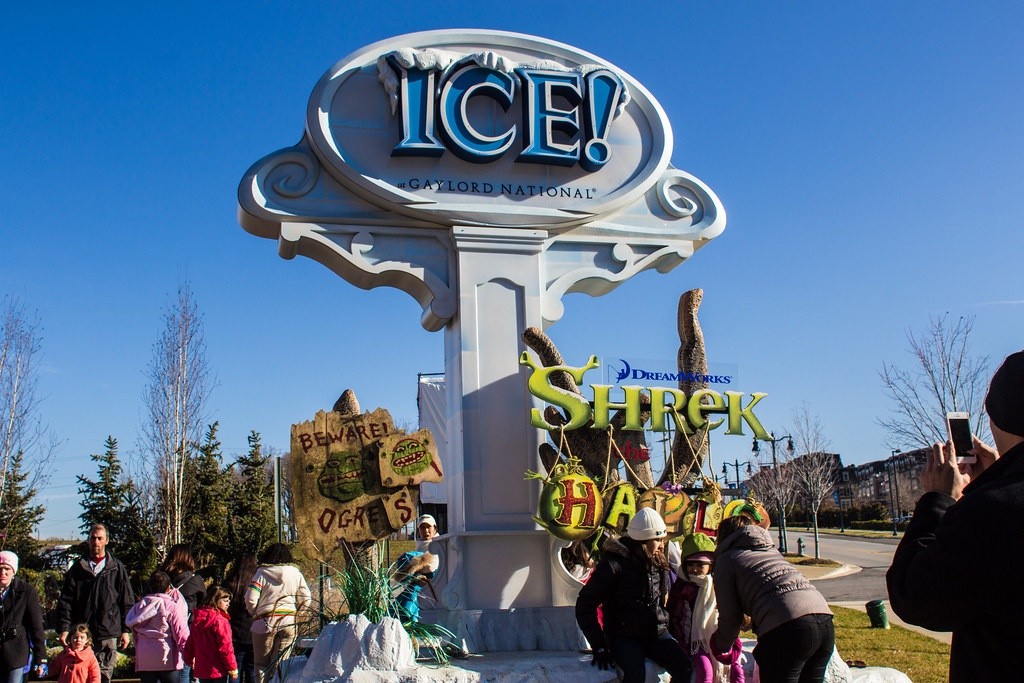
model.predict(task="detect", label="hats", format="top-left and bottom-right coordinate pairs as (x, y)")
top-left (418, 514), bottom-right (436, 527)
top-left (681, 532), bottom-right (715, 563)
top-left (0, 551), bottom-right (18, 574)
top-left (985, 350), bottom-right (1024, 437)
top-left (628, 507), bottom-right (667, 540)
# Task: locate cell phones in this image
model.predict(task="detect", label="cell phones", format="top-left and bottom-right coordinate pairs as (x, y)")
top-left (945, 412), bottom-right (976, 464)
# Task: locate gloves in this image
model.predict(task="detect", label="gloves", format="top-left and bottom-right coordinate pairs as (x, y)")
top-left (591, 647), bottom-right (616, 670)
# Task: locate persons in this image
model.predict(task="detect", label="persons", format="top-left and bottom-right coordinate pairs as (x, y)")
top-left (182, 586), bottom-right (238, 683)
top-left (710, 515), bottom-right (834, 683)
top-left (0, 551), bottom-right (47, 683)
top-left (666, 532), bottom-right (745, 683)
top-left (37, 624), bottom-right (101, 683)
top-left (160, 544), bottom-right (207, 683)
top-left (574, 507), bottom-right (693, 683)
top-left (222, 551), bottom-right (257, 683)
top-left (125, 569), bottom-right (190, 683)
top-left (385, 550), bottom-right (439, 623)
top-left (886, 350), bottom-right (1024, 683)
top-left (416, 514), bottom-right (439, 540)
top-left (244, 542), bottom-right (312, 683)
top-left (54, 524), bottom-right (135, 683)
